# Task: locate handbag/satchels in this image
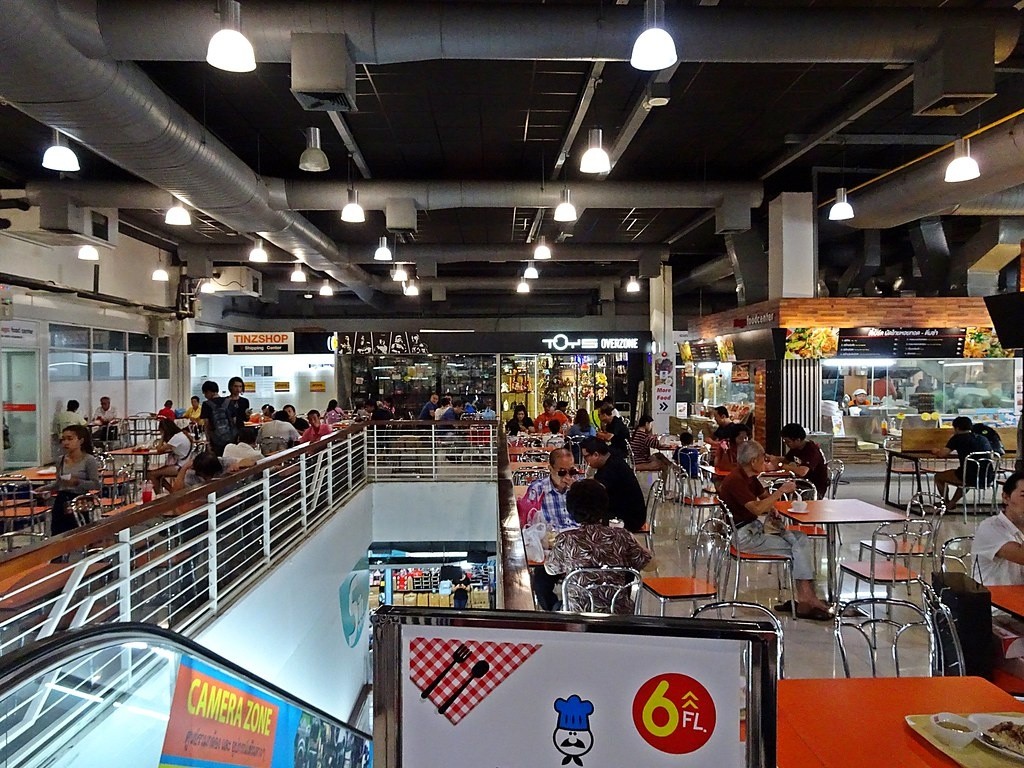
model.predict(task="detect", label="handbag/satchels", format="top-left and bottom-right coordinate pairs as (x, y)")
top-left (63, 497), bottom-right (95, 513)
top-left (518, 489), bottom-right (544, 529)
top-left (522, 508), bottom-right (546, 564)
top-left (930, 552), bottom-right (994, 681)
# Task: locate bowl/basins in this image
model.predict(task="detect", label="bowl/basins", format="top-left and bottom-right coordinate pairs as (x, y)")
top-left (791, 502), bottom-right (807, 511)
top-left (930, 712), bottom-right (978, 749)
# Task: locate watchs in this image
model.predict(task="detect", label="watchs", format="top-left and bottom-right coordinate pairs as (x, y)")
top-left (777, 461), bottom-right (784, 468)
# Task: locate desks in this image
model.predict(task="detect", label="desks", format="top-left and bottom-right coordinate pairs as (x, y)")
top-left (0, 467), bottom-right (57, 483)
top-left (697, 464), bottom-right (792, 478)
top-left (657, 447), bottom-right (701, 451)
top-left (162, 497), bottom-right (240, 521)
top-left (509, 445), bottom-right (554, 456)
top-left (0, 562), bottom-right (113, 613)
top-left (884, 448), bottom-right (1016, 517)
top-left (109, 446), bottom-right (172, 481)
top-left (511, 462), bottom-right (550, 469)
top-left (741, 675), bottom-right (1024, 768)
top-left (775, 498), bottom-right (907, 618)
top-left (983, 585), bottom-right (1024, 623)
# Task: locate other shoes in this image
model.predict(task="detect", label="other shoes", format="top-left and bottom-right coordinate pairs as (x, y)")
top-left (934, 500), bottom-right (950, 507)
top-left (445, 455), bottom-right (459, 463)
top-left (666, 489), bottom-right (680, 499)
top-left (947, 502), bottom-right (956, 510)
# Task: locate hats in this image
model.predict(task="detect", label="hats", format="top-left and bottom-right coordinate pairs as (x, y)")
top-left (854, 389), bottom-right (867, 396)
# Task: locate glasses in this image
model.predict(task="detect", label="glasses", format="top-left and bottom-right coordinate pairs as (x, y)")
top-left (558, 469), bottom-right (577, 476)
top-left (584, 454), bottom-right (591, 462)
top-left (783, 439), bottom-right (792, 444)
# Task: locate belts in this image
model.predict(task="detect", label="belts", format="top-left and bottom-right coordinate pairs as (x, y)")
top-left (728, 518), bottom-right (755, 537)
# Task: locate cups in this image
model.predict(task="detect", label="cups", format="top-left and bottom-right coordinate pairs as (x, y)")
top-left (140, 484), bottom-right (153, 504)
top-left (609, 520), bottom-right (624, 529)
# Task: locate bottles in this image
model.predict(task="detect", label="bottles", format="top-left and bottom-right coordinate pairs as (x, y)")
top-left (665, 428), bottom-right (670, 445)
top-left (688, 427), bottom-right (692, 434)
top-left (698, 432), bottom-right (703, 446)
top-left (538, 423), bottom-right (542, 433)
top-left (881, 418), bottom-right (888, 436)
top-left (890, 418), bottom-right (896, 434)
top-left (562, 423), bottom-right (567, 436)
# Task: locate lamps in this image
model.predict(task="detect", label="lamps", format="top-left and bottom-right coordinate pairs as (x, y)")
top-left (43, 0), bottom-right (982, 296)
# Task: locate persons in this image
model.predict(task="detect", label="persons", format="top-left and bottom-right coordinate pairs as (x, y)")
top-left (1015, 413), bottom-right (1024, 470)
top-left (149, 377), bottom-right (348, 601)
top-left (933, 416), bottom-right (996, 509)
top-left (418, 394), bottom-right (466, 464)
top-left (765, 422), bottom-right (829, 526)
top-left (308, 718), bottom-right (369, 768)
top-left (970, 472), bottom-right (1024, 616)
top-left (505, 399), bottom-right (630, 460)
top-left (526, 449), bottom-right (581, 611)
top-left (545, 479), bottom-right (651, 615)
top-left (672, 432), bottom-right (702, 497)
top-left (34, 396), bottom-right (122, 563)
top-left (847, 388), bottom-right (870, 407)
top-left (580, 436), bottom-right (647, 530)
top-left (874, 370), bottom-right (897, 399)
top-left (630, 414), bottom-right (672, 499)
top-left (364, 395), bottom-right (411, 460)
top-left (705, 406), bottom-right (752, 536)
top-left (718, 440), bottom-right (835, 621)
top-left (452, 574), bottom-right (471, 608)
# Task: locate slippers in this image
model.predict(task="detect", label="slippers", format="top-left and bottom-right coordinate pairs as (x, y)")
top-left (774, 600), bottom-right (799, 614)
top-left (796, 607), bottom-right (835, 621)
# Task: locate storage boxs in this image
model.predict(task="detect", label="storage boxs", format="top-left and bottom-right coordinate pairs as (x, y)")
top-left (369, 571), bottom-right (490, 609)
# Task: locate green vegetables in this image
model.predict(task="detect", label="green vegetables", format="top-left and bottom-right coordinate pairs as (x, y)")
top-left (791, 328), bottom-right (826, 350)
top-left (972, 332), bottom-right (1004, 359)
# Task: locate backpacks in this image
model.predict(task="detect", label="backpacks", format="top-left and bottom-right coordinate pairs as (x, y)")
top-left (972, 422), bottom-right (1006, 456)
top-left (207, 397), bottom-right (236, 446)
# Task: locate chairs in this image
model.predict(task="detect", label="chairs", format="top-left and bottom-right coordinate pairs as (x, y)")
top-left (495, 412), bottom-right (1024, 678)
top-left (0, 407), bottom-right (491, 716)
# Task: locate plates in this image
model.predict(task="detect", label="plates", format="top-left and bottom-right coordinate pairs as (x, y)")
top-left (964, 712), bottom-right (1024, 762)
top-left (787, 509), bottom-right (809, 514)
top-left (36, 469), bottom-right (56, 474)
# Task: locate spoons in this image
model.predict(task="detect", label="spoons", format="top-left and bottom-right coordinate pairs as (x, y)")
top-left (794, 491), bottom-right (802, 502)
